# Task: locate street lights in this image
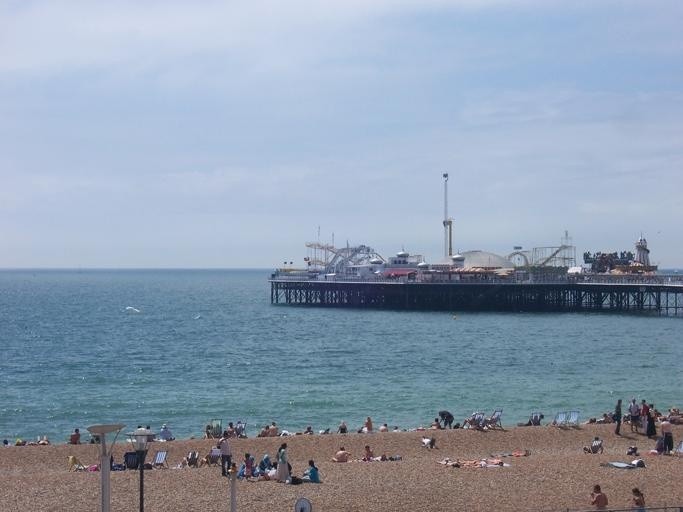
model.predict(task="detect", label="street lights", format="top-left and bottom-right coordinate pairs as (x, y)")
top-left (124, 427), bottom-right (159, 512)
top-left (86, 424), bottom-right (126, 511)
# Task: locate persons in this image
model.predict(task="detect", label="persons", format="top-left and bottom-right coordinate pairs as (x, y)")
top-left (582, 436), bottom-right (603, 454)
top-left (218, 431), bottom-right (232, 475)
top-left (138, 424), bottom-right (174, 441)
top-left (205, 425), bottom-right (214, 439)
top-left (228, 421), bottom-right (246, 437)
top-left (258, 423), bottom-right (293, 437)
top-left (630, 487), bottom-right (645, 512)
top-left (422, 411), bottom-right (460, 467)
top-left (238, 443), bottom-right (319, 482)
top-left (332, 445), bottom-right (386, 462)
top-left (70, 429), bottom-right (80, 445)
top-left (303, 421), bottom-right (348, 434)
top-left (590, 485), bottom-right (608, 512)
top-left (611, 399), bottom-right (673, 455)
top-left (361, 417), bottom-right (401, 433)
top-left (179, 445), bottom-right (220, 467)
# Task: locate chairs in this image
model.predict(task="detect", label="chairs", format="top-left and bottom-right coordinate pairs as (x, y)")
top-left (203, 449), bottom-right (223, 467)
top-left (151, 450), bottom-right (169, 469)
top-left (468, 412), bottom-right (484, 430)
top-left (67, 455), bottom-right (86, 472)
top-left (554, 411), bottom-right (568, 424)
top-left (210, 418), bottom-right (222, 438)
top-left (123, 452), bottom-right (139, 469)
top-left (567, 411), bottom-right (580, 425)
top-left (672, 441), bottom-right (683, 464)
top-left (485, 410), bottom-right (505, 431)
top-left (234, 423), bottom-right (247, 440)
